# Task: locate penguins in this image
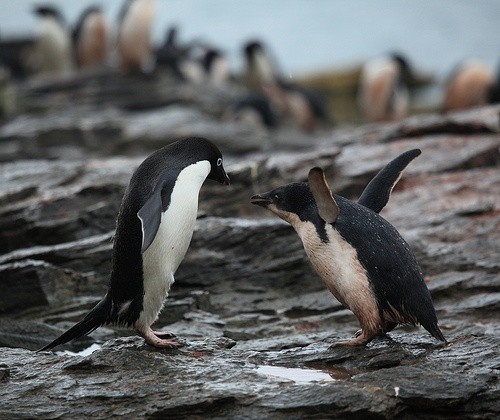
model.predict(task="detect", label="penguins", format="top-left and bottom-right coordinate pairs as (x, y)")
top-left (71, 6), bottom-right (110, 66)
top-left (34, 4), bottom-right (75, 67)
top-left (199, 40), bottom-right (228, 87)
top-left (157, 27), bottom-right (180, 62)
top-left (347, 52), bottom-right (412, 121)
top-left (249, 148), bottom-right (447, 348)
top-left (247, 41), bottom-right (279, 87)
top-left (273, 74), bottom-right (334, 129)
top-left (115, 0), bottom-right (160, 70)
top-left (438, 54), bottom-right (499, 111)
top-left (173, 36), bottom-right (208, 82)
top-left (36, 136), bottom-right (231, 351)
top-left (387, 77), bottom-right (411, 120)
